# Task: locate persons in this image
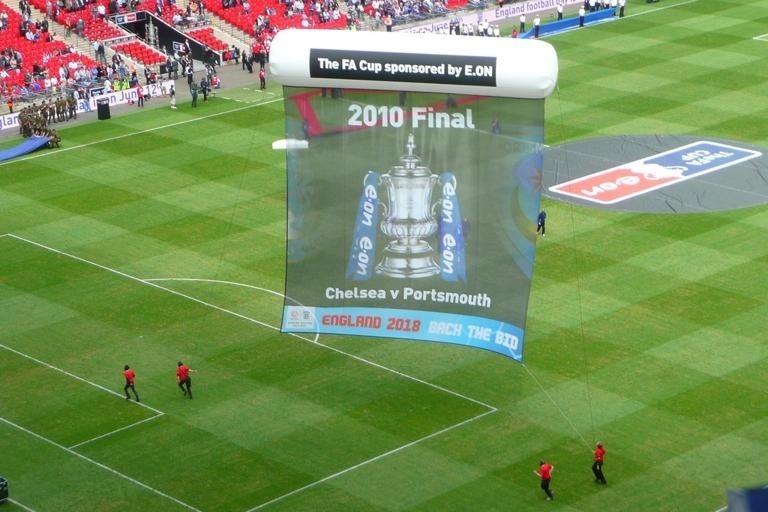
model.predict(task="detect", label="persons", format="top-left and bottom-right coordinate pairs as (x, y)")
top-left (122, 365), bottom-right (140, 403)
top-left (535, 210), bottom-right (547, 237)
top-left (533, 458), bottom-right (557, 501)
top-left (275, 1), bottom-right (501, 40)
top-left (520, 0), bottom-right (626, 38)
top-left (0, 0), bottom-right (275, 109)
top-left (17, 96), bottom-right (77, 149)
top-left (177, 361), bottom-right (198, 401)
top-left (590, 441), bottom-right (607, 485)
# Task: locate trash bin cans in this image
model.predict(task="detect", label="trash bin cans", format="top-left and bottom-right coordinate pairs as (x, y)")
top-left (97, 97), bottom-right (109, 120)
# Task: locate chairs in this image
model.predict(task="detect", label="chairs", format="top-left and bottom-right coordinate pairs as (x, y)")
top-left (0, 0), bottom-right (350, 97)
